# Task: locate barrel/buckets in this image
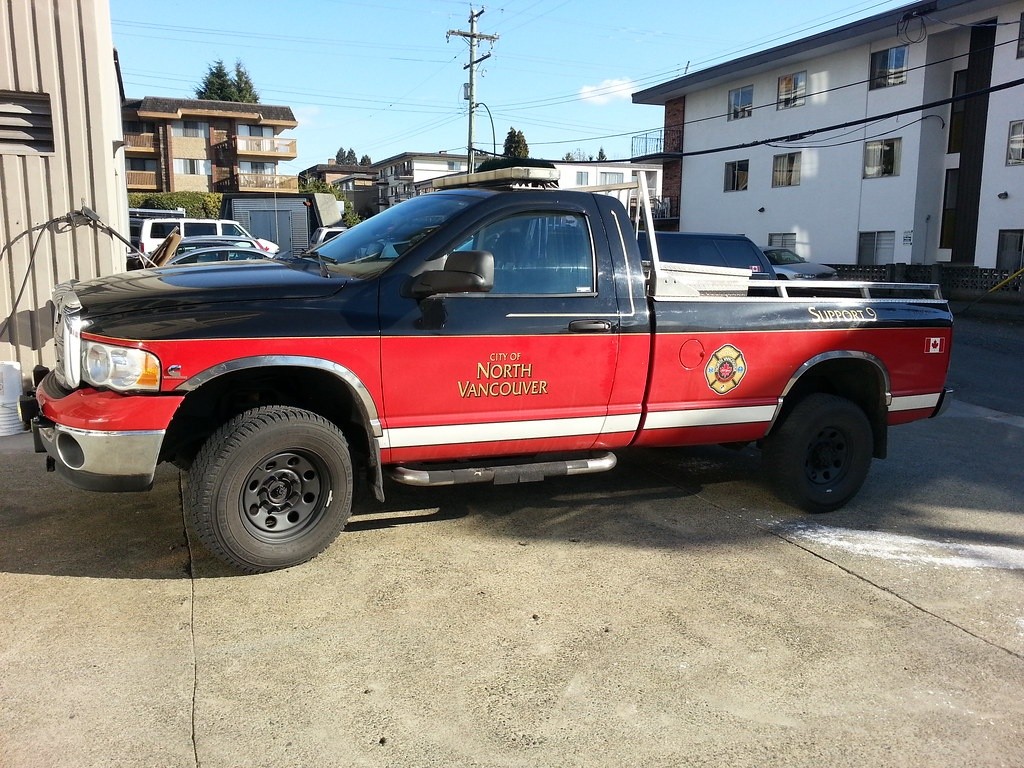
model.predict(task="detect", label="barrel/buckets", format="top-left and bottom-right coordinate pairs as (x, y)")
top-left (0, 399), bottom-right (25, 436)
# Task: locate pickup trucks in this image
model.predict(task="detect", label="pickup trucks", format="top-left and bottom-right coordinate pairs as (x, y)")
top-left (16, 167), bottom-right (955, 573)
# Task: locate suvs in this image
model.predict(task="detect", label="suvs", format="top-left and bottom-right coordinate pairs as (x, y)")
top-left (310, 226), bottom-right (347, 249)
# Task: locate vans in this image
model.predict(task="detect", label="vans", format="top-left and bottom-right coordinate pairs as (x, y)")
top-left (138, 217), bottom-right (253, 258)
top-left (632, 229), bottom-right (777, 281)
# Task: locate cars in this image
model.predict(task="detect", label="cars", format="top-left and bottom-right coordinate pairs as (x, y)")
top-left (154, 235), bottom-right (278, 267)
top-left (758, 246), bottom-right (839, 280)
